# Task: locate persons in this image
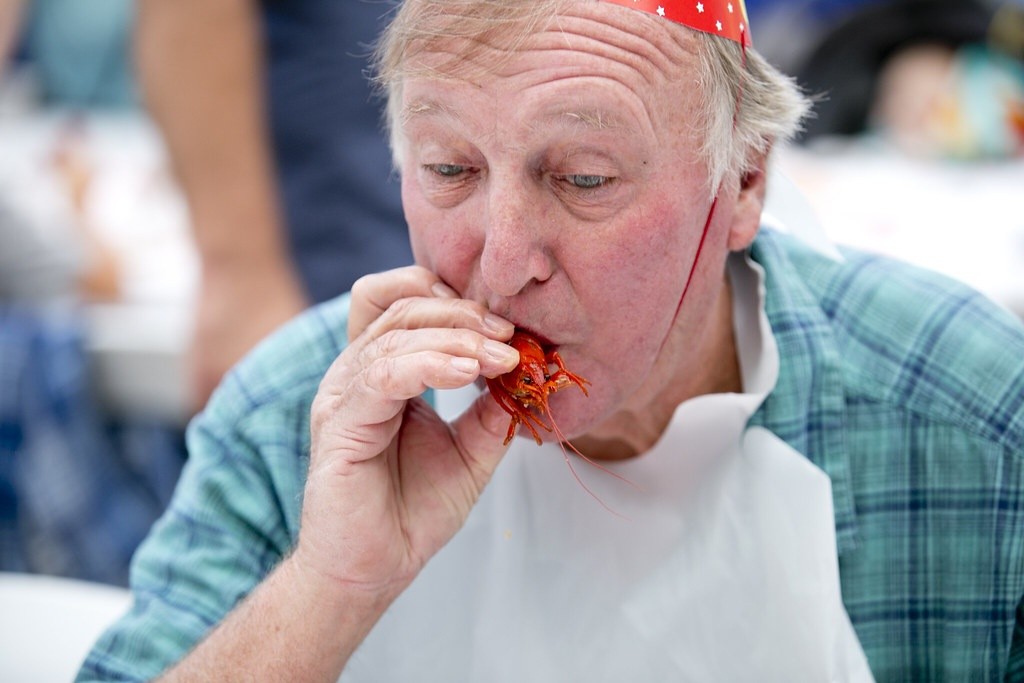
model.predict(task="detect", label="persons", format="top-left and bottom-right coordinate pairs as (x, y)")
top-left (0, 0), bottom-right (412, 590)
top-left (76, 0), bottom-right (1024, 683)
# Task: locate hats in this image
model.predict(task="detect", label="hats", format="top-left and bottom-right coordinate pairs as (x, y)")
top-left (604, 0), bottom-right (752, 47)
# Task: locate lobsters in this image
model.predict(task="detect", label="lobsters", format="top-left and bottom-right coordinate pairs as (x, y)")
top-left (486, 331), bottom-right (638, 521)
